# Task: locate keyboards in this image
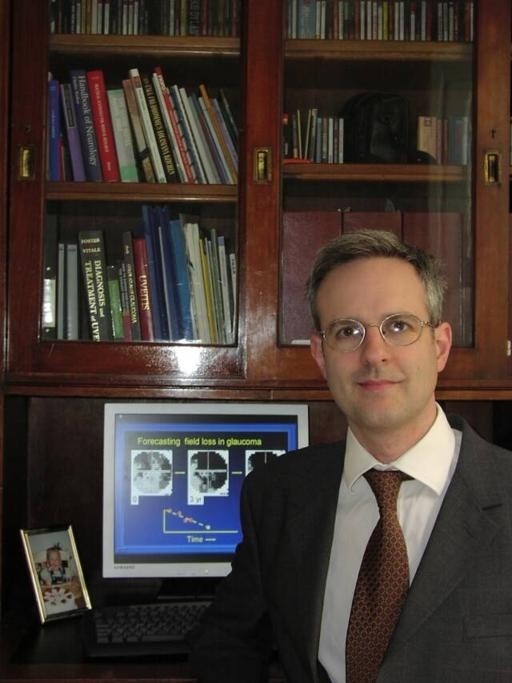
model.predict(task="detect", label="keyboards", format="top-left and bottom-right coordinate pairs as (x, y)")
top-left (81, 600), bottom-right (213, 660)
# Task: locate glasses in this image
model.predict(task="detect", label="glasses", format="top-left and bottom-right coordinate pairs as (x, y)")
top-left (316, 310), bottom-right (435, 353)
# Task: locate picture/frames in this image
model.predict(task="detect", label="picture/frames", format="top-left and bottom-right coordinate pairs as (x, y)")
top-left (20, 524), bottom-right (94, 625)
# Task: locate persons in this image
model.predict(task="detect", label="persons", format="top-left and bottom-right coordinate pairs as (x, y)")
top-left (37, 543), bottom-right (73, 583)
top-left (186, 227), bottom-right (510, 682)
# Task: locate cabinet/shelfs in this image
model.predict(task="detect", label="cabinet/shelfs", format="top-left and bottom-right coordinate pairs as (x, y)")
top-left (0, 0), bottom-right (512, 404)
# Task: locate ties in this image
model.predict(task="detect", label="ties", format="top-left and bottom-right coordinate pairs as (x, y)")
top-left (346, 467), bottom-right (415, 682)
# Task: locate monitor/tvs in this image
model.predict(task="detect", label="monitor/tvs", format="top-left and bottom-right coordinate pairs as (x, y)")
top-left (101, 402), bottom-right (310, 602)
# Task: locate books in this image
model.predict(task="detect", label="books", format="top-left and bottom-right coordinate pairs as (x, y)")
top-left (440, 117), bottom-right (473, 165)
top-left (40, 205), bottom-right (239, 342)
top-left (48, 2), bottom-right (241, 36)
top-left (42, 66), bottom-right (240, 184)
top-left (285, 2), bottom-right (474, 42)
top-left (281, 109), bottom-right (344, 165)
